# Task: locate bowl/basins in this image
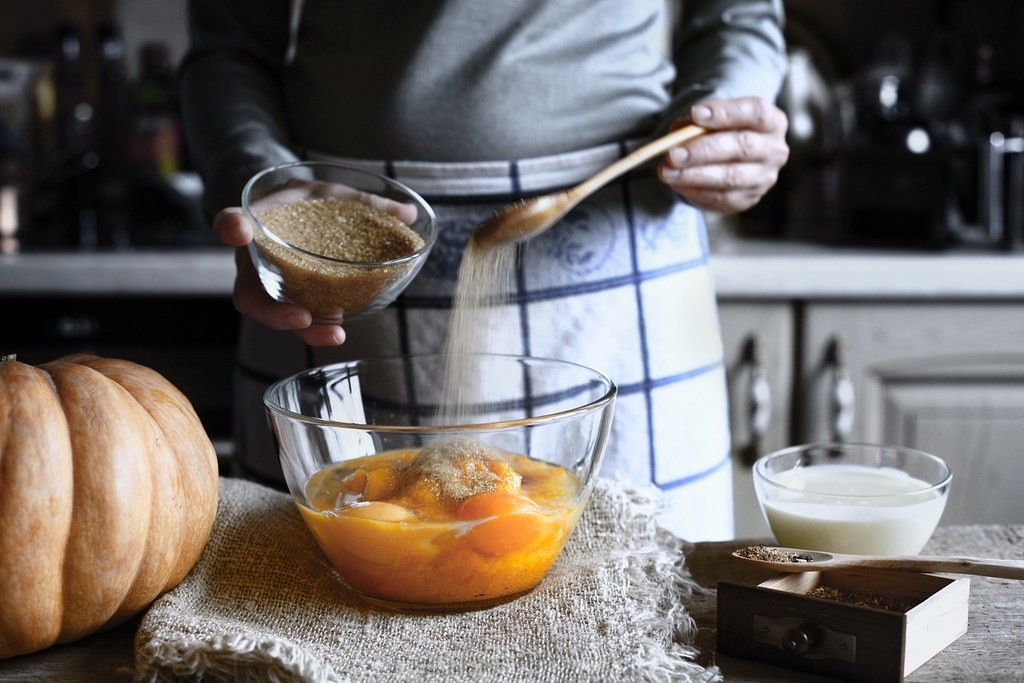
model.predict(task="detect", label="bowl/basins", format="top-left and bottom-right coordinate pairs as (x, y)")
top-left (242, 161), bottom-right (438, 324)
top-left (263, 352), bottom-right (619, 612)
top-left (750, 441), bottom-right (953, 556)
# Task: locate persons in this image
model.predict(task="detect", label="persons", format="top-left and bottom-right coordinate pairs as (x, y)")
top-left (184, 0), bottom-right (788, 543)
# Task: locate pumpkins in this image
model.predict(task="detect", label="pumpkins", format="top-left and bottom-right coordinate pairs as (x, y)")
top-left (0, 348), bottom-right (220, 660)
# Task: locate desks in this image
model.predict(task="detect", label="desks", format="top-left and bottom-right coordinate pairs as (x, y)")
top-left (0, 523), bottom-right (1024, 683)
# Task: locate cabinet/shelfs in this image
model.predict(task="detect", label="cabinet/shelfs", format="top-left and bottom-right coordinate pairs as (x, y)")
top-left (0, 205), bottom-right (1024, 524)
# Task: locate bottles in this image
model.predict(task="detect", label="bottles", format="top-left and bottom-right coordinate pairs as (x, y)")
top-left (48, 40), bottom-right (180, 191)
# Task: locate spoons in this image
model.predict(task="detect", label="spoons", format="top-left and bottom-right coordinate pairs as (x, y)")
top-left (470, 125), bottom-right (705, 249)
top-left (731, 545), bottom-right (1024, 583)
